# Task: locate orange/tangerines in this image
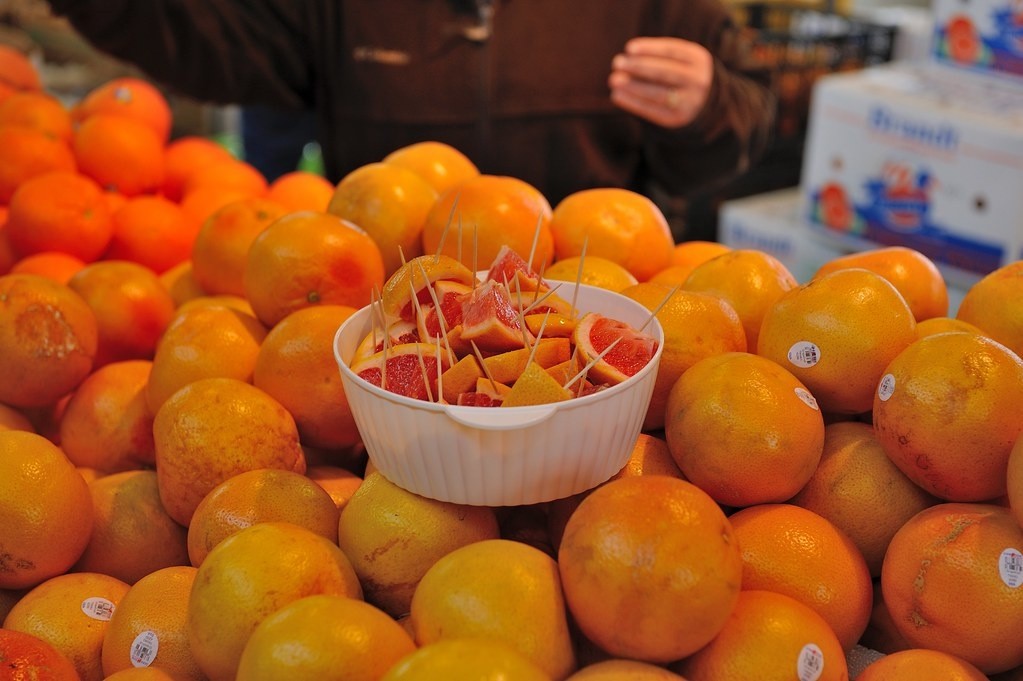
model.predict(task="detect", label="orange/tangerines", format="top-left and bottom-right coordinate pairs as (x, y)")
top-left (0, 45), bottom-right (1023, 681)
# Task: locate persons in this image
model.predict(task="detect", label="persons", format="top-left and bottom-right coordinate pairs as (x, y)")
top-left (42, 1), bottom-right (783, 253)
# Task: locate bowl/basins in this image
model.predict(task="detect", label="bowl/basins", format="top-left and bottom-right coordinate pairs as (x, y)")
top-left (333, 270), bottom-right (665, 507)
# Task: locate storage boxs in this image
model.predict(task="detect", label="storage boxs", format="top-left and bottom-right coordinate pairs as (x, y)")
top-left (719, 0), bottom-right (1023, 322)
